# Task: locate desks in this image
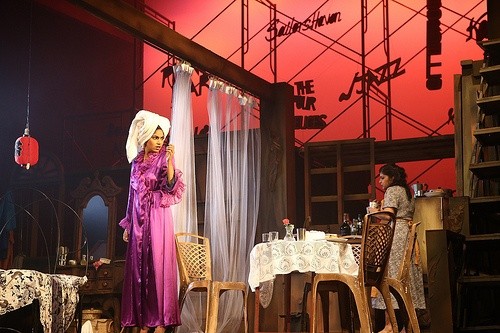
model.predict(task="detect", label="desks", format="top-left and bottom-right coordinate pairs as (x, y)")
top-left (248, 238), bottom-right (359, 333)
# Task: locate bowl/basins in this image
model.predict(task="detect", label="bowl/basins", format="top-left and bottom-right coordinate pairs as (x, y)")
top-left (69, 260), bottom-right (77, 265)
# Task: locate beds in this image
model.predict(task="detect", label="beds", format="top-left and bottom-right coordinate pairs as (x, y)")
top-left (0, 267), bottom-right (89, 333)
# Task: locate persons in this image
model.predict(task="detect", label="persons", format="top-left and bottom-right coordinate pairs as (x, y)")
top-left (366, 163), bottom-right (426, 333)
top-left (121, 110), bottom-right (186, 333)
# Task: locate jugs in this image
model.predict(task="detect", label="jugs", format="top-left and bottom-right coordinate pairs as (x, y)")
top-left (411, 181), bottom-right (429, 198)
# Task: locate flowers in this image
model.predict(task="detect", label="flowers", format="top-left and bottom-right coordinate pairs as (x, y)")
top-left (282, 219), bottom-right (290, 228)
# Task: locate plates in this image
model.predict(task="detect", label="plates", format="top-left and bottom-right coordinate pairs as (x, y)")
top-left (424, 192), bottom-right (446, 197)
top-left (326, 238), bottom-right (348, 242)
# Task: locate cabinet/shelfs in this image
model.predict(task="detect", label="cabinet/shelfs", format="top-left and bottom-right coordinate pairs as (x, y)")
top-left (425, 32), bottom-right (500, 333)
top-left (46, 260), bottom-right (126, 333)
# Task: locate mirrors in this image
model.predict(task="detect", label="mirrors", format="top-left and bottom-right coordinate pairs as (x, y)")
top-left (67, 166), bottom-right (125, 262)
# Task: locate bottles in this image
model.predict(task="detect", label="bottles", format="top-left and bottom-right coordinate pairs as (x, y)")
top-left (340, 213), bottom-right (364, 236)
top-left (90, 255), bottom-right (93, 260)
top-left (81, 254), bottom-right (87, 265)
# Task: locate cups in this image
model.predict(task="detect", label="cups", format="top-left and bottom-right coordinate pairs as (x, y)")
top-left (269, 231), bottom-right (279, 242)
top-left (297, 228), bottom-right (305, 241)
top-left (262, 233), bottom-right (269, 242)
top-left (418, 189), bottom-right (424, 196)
top-left (370, 202), bottom-right (378, 209)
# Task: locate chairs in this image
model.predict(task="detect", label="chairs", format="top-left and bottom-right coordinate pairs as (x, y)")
top-left (173, 232), bottom-right (248, 333)
top-left (340, 219), bottom-right (423, 333)
top-left (309, 210), bottom-right (398, 333)
